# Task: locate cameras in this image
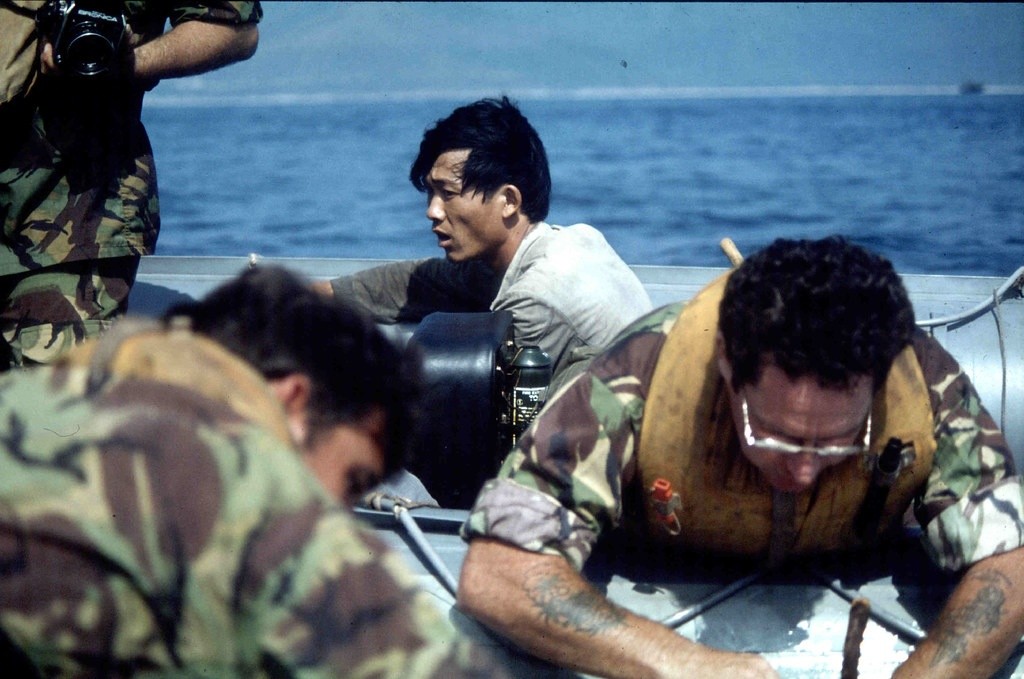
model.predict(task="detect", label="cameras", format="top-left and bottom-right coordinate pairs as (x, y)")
top-left (42, 0), bottom-right (127, 78)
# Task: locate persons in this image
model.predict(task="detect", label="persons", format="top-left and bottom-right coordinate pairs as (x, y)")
top-left (455, 232), bottom-right (1023, 679)
top-left (309, 95), bottom-right (660, 378)
top-left (2, 1), bottom-right (514, 679)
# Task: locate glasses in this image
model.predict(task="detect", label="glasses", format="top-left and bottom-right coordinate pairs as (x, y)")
top-left (741, 394), bottom-right (872, 458)
